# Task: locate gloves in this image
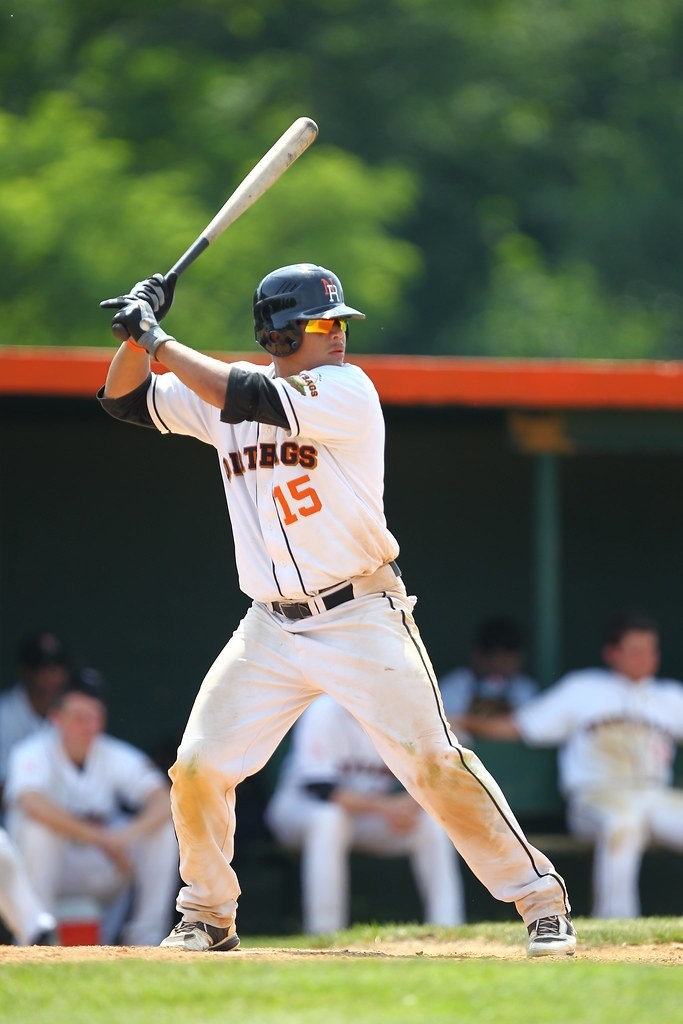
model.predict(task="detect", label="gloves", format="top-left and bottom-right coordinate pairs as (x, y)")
top-left (129, 273), bottom-right (178, 325)
top-left (99, 295), bottom-right (176, 362)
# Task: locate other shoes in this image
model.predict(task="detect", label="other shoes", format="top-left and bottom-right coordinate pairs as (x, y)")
top-left (19, 914), bottom-right (56, 944)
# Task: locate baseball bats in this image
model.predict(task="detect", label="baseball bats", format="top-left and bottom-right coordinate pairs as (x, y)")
top-left (111, 117), bottom-right (317, 341)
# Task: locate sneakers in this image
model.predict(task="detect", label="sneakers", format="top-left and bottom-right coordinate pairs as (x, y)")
top-left (528, 912), bottom-right (576, 956)
top-left (160, 920), bottom-right (240, 951)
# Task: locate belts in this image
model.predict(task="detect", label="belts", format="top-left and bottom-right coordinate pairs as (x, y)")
top-left (272, 560), bottom-right (402, 620)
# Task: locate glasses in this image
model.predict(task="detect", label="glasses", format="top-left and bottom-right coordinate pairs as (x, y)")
top-left (297, 318), bottom-right (347, 335)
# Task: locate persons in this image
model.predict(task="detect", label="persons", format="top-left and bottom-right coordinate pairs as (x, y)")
top-left (438, 616), bottom-right (540, 736)
top-left (0, 635), bottom-right (180, 948)
top-left (265, 692), bottom-right (468, 937)
top-left (95, 264), bottom-right (577, 960)
top-left (446, 624), bottom-right (682, 921)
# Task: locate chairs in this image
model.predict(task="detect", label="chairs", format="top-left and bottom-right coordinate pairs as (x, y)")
top-left (469, 739), bottom-right (593, 917)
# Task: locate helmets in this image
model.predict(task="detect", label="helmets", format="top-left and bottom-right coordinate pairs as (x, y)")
top-left (253, 263), bottom-right (366, 357)
